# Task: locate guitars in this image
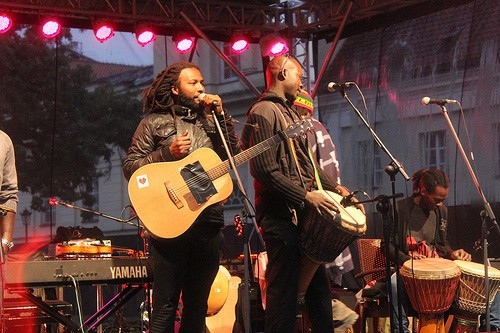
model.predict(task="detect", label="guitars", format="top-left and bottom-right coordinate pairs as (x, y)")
top-left (126, 115), bottom-right (315, 243)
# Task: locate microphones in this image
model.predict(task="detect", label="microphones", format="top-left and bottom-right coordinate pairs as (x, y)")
top-left (48, 196), bottom-right (71, 207)
top-left (421, 96), bottom-right (457, 106)
top-left (327, 82), bottom-right (356, 92)
top-left (198, 92), bottom-right (220, 106)
top-left (340, 190), bottom-right (359, 206)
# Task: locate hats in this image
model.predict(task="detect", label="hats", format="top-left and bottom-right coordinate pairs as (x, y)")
top-left (292, 89), bottom-right (314, 111)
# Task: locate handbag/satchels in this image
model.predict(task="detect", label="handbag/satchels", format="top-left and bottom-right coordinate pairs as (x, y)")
top-left (55, 226), bottom-right (104, 244)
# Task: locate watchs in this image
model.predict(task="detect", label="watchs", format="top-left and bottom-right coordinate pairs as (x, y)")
top-left (2, 238), bottom-right (14, 252)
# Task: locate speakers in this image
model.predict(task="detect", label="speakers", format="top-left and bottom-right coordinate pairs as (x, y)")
top-left (222, 223), bottom-right (266, 260)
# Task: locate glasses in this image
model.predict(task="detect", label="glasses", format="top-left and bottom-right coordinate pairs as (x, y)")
top-left (280, 52), bottom-right (294, 72)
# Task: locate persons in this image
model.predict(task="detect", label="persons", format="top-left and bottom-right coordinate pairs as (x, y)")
top-left (240, 52), bottom-right (366, 333)
top-left (122, 61), bottom-right (241, 333)
top-left (0, 131), bottom-right (19, 316)
top-left (380, 168), bottom-right (500, 333)
top-left (291, 90), bottom-right (354, 278)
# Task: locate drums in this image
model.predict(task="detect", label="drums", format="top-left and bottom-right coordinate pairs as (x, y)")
top-left (453, 258), bottom-right (500, 332)
top-left (397, 256), bottom-right (461, 333)
top-left (294, 189), bottom-right (367, 264)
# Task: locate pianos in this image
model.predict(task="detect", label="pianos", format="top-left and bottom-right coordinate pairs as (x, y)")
top-left (5, 250), bottom-right (150, 288)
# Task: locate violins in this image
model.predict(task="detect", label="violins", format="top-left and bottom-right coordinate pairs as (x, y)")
top-left (54, 240), bottom-right (145, 258)
